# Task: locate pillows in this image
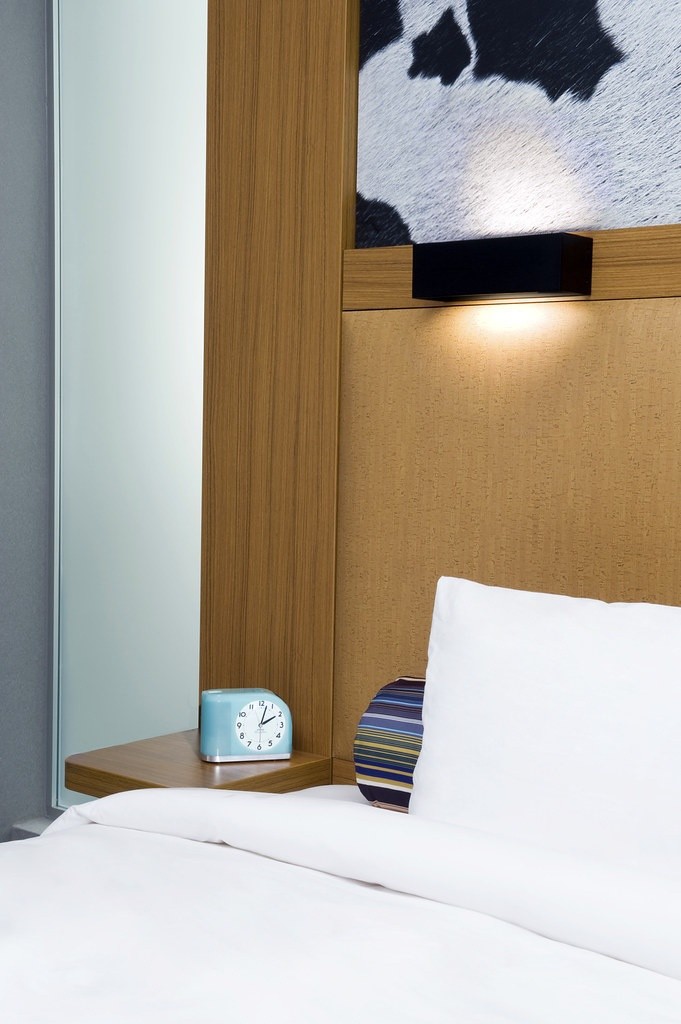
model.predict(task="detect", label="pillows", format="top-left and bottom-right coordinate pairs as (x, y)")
top-left (351, 674), bottom-right (423, 813)
top-left (406, 576), bottom-right (681, 824)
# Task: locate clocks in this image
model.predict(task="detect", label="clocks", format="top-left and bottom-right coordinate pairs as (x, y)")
top-left (236, 700), bottom-right (285, 751)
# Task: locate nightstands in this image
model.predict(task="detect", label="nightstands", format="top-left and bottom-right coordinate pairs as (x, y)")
top-left (64, 728), bottom-right (331, 799)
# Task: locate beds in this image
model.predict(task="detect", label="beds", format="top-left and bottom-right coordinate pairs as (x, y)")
top-left (0, 784), bottom-right (678, 1024)
